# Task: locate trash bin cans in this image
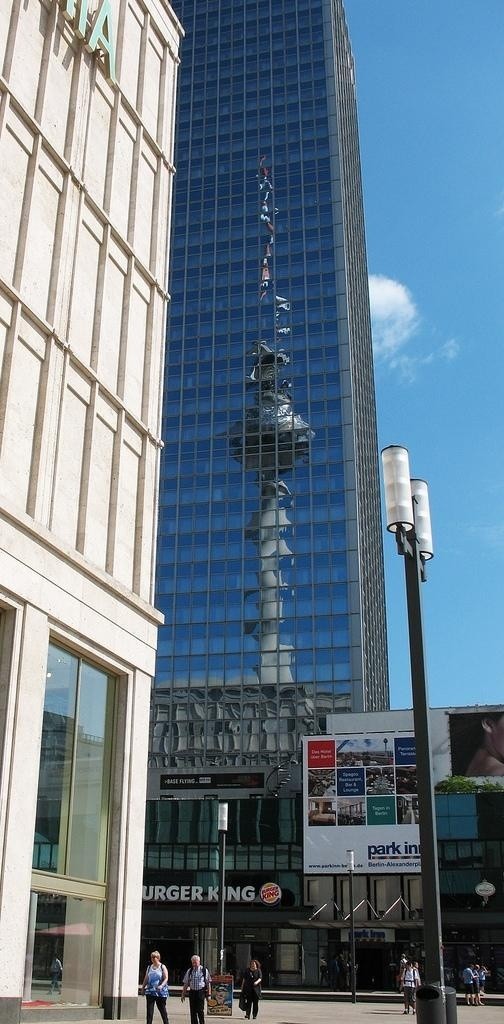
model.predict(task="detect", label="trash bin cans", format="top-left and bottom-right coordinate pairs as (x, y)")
top-left (415, 984), bottom-right (457, 1024)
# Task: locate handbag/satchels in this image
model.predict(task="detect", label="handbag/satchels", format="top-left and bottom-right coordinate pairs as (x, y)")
top-left (239, 991), bottom-right (248, 1011)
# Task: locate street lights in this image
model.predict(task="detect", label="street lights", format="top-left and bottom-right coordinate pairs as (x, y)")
top-left (378, 443), bottom-right (460, 1022)
top-left (345, 849), bottom-right (356, 1004)
top-left (215, 802), bottom-right (229, 975)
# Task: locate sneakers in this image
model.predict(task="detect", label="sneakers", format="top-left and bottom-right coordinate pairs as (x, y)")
top-left (245, 1014), bottom-right (256, 1019)
top-left (403, 1010), bottom-right (416, 1015)
top-left (465, 1000), bottom-right (485, 1006)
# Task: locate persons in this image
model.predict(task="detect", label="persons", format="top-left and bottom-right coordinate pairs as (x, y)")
top-left (238, 959), bottom-right (264, 1020)
top-left (180, 954), bottom-right (212, 1024)
top-left (464, 711), bottom-right (504, 776)
top-left (319, 949), bottom-right (360, 989)
top-left (43, 953), bottom-right (63, 997)
top-left (141, 950), bottom-right (169, 1024)
top-left (395, 953), bottom-right (488, 1015)
